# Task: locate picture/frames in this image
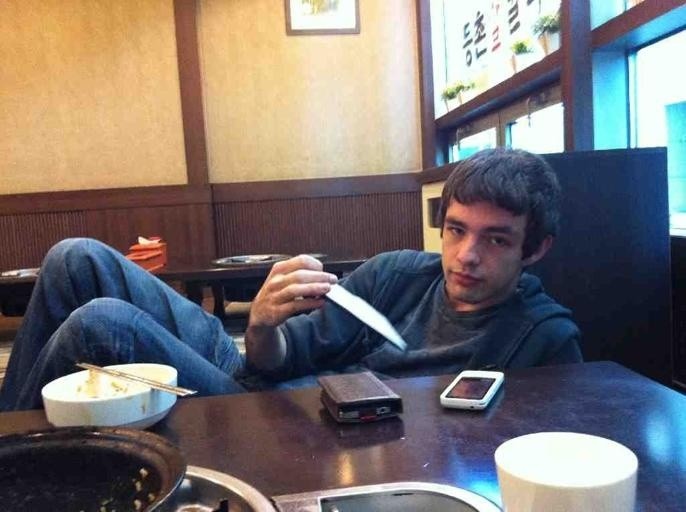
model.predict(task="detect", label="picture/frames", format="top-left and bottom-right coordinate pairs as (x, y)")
top-left (285, 0), bottom-right (360, 35)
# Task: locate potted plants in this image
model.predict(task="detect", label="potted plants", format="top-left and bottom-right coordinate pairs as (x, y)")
top-left (530, 15), bottom-right (562, 56)
top-left (441, 82), bottom-right (477, 113)
top-left (512, 39), bottom-right (536, 73)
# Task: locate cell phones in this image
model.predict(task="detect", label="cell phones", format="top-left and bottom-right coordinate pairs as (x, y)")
top-left (440, 370), bottom-right (505, 412)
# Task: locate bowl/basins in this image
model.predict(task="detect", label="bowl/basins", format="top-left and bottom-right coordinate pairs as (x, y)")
top-left (42, 362), bottom-right (177, 437)
top-left (0, 268), bottom-right (43, 317)
top-left (210, 254), bottom-right (294, 303)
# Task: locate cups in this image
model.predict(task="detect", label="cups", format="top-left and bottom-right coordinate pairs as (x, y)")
top-left (495, 432), bottom-right (636, 512)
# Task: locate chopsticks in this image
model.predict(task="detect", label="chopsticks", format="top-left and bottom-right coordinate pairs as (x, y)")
top-left (75, 361), bottom-right (198, 398)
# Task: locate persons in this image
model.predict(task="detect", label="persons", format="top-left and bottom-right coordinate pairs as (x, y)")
top-left (1, 147), bottom-right (586, 410)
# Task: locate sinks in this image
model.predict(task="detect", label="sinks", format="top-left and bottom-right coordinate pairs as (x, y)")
top-left (272, 480), bottom-right (505, 512)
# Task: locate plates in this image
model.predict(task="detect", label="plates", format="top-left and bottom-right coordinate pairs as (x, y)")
top-left (1, 425), bottom-right (186, 512)
